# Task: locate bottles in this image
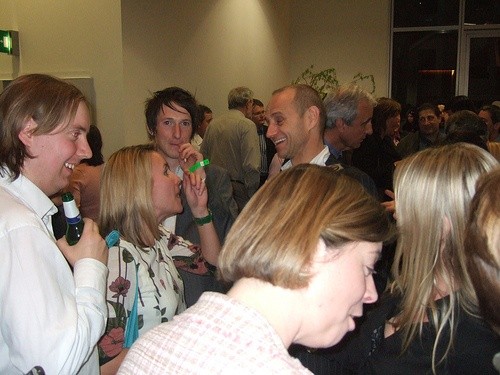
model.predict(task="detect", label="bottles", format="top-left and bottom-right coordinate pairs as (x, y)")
top-left (61, 192), bottom-right (87, 273)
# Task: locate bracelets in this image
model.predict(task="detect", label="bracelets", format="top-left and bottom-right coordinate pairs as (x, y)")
top-left (190, 208), bottom-right (214, 225)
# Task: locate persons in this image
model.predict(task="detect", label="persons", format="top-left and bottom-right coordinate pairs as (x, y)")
top-left (370, 90), bottom-right (500, 375)
top-left (201, 87), bottom-right (260, 207)
top-left (52, 123), bottom-right (104, 228)
top-left (400, 103), bottom-right (447, 156)
top-left (140, 87), bottom-right (239, 308)
top-left (117, 163), bottom-right (397, 374)
top-left (265, 85), bottom-right (380, 348)
top-left (241, 99), bottom-right (279, 177)
top-left (1, 73), bottom-right (108, 375)
top-left (185, 104), bottom-right (213, 152)
top-left (312, 86), bottom-right (378, 167)
top-left (90, 144), bottom-right (222, 375)
